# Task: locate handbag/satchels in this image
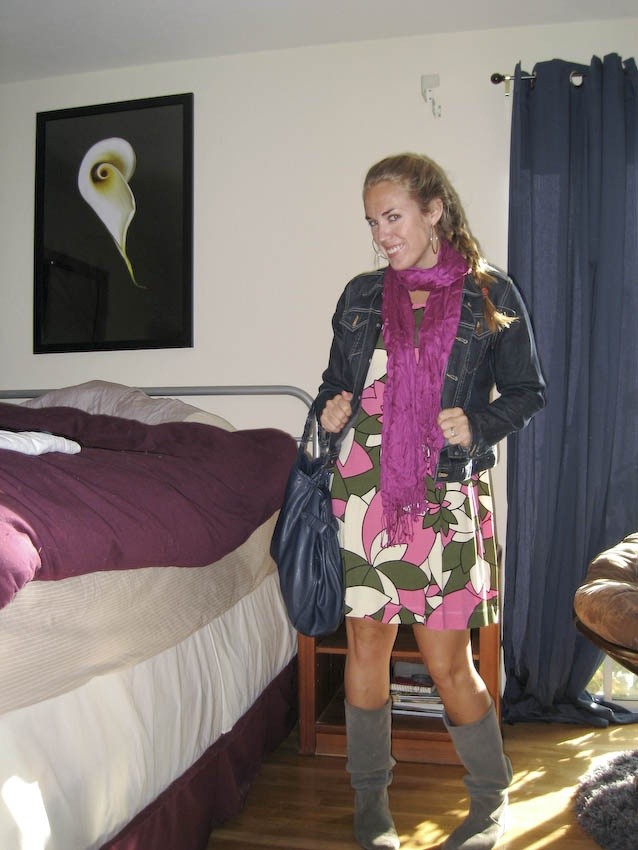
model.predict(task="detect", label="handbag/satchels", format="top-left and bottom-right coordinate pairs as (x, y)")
top-left (269, 387), bottom-right (352, 637)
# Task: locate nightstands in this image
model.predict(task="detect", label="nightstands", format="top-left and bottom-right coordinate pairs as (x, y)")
top-left (294, 541), bottom-right (504, 765)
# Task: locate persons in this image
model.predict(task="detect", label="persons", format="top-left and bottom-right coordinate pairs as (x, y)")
top-left (315, 154), bottom-right (547, 850)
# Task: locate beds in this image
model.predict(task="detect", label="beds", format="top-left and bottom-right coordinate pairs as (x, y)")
top-left (0, 378), bottom-right (315, 850)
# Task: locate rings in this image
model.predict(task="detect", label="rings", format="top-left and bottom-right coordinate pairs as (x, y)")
top-left (451, 427), bottom-right (456, 438)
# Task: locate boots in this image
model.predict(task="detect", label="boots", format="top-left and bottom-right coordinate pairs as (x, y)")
top-left (345, 694), bottom-right (401, 850)
top-left (440, 697), bottom-right (514, 850)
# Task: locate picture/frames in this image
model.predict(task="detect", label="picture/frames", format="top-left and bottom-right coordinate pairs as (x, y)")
top-left (31, 91), bottom-right (196, 354)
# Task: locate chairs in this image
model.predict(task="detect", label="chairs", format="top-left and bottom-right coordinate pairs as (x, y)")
top-left (570, 528), bottom-right (638, 678)
